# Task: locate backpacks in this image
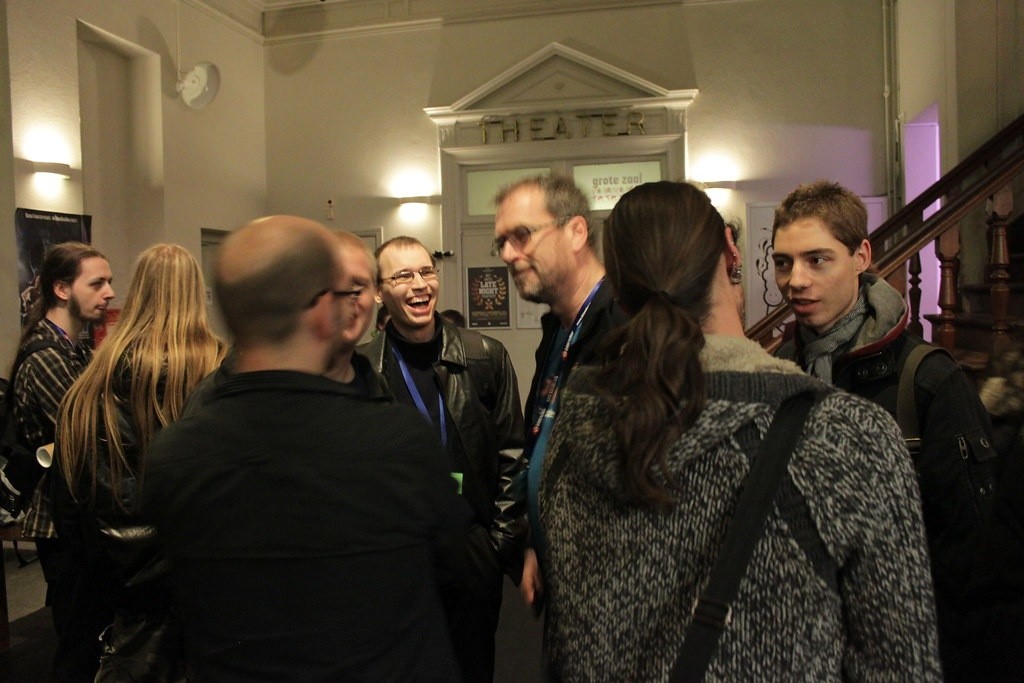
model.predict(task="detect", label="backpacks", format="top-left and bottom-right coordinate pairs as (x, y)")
top-left (0, 339), bottom-right (71, 520)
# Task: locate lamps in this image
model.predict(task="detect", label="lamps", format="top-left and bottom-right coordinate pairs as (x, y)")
top-left (169, 0), bottom-right (222, 110)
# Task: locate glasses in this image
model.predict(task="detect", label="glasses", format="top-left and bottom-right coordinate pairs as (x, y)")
top-left (306, 285), bottom-right (366, 308)
top-left (380, 266), bottom-right (440, 284)
top-left (490, 216), bottom-right (574, 257)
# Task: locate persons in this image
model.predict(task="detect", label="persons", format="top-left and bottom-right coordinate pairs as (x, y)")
top-left (323, 226), bottom-right (398, 404)
top-left (140, 214), bottom-right (460, 683)
top-left (439, 309), bottom-right (464, 329)
top-left (538, 182), bottom-right (946, 683)
top-left (0, 242), bottom-right (115, 683)
top-left (369, 307), bottom-right (392, 340)
top-left (43, 242), bottom-right (230, 611)
top-left (768, 183), bottom-right (997, 683)
top-left (489, 173), bottom-right (613, 612)
top-left (354, 236), bottom-right (526, 683)
